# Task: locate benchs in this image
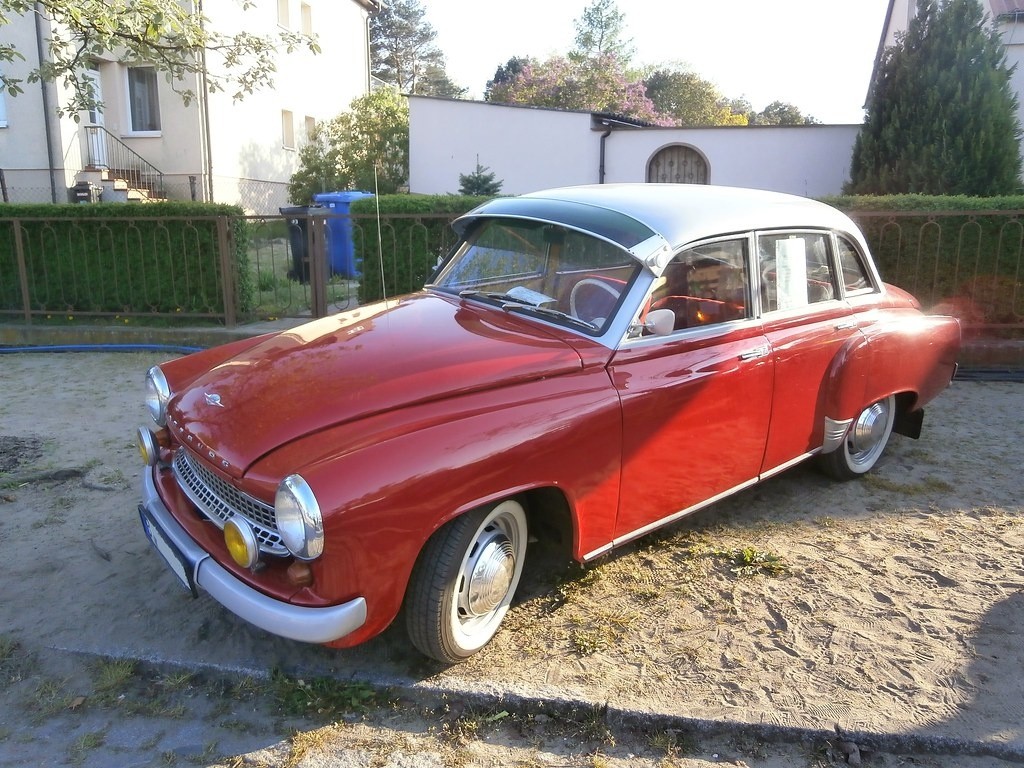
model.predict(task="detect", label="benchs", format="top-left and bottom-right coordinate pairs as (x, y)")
top-left (716, 267), bottom-right (839, 314)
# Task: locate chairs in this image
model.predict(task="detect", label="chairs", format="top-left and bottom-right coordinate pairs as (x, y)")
top-left (644, 295), bottom-right (749, 336)
top-left (558, 275), bottom-right (649, 333)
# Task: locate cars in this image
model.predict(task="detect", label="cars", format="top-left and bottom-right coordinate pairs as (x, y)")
top-left (134, 181), bottom-right (965, 663)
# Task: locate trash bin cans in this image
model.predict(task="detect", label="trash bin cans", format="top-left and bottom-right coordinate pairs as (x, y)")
top-left (278, 204), bottom-right (322, 283)
top-left (312, 190), bottom-right (376, 280)
